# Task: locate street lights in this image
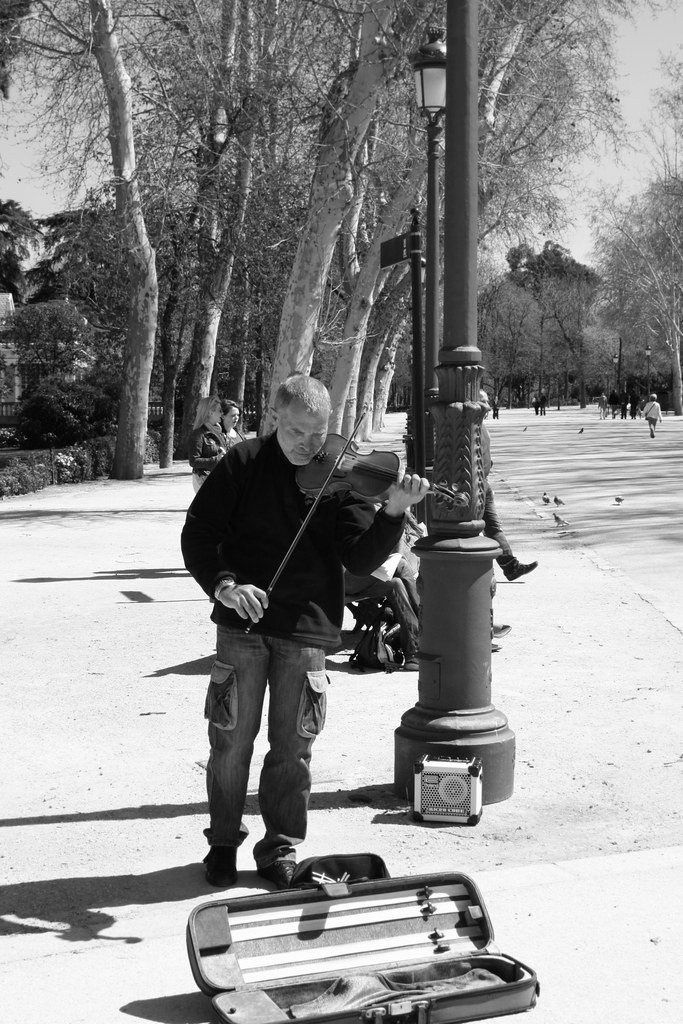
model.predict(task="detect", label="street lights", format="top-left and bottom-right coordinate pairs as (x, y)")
top-left (645, 345), bottom-right (651, 402)
top-left (612, 336), bottom-right (622, 390)
top-left (404, 19), bottom-right (470, 483)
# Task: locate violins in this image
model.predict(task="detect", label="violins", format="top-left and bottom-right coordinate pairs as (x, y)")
top-left (295, 432), bottom-right (470, 512)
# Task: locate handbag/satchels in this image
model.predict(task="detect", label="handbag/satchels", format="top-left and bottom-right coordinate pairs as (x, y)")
top-left (291, 853), bottom-right (393, 890)
top-left (355, 602), bottom-right (406, 672)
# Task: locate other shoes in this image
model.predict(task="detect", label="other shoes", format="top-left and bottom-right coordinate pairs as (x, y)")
top-left (402, 658), bottom-right (419, 672)
top-left (258, 857), bottom-right (298, 887)
top-left (491, 625), bottom-right (512, 651)
top-left (503, 556), bottom-right (538, 581)
top-left (205, 845), bottom-right (238, 887)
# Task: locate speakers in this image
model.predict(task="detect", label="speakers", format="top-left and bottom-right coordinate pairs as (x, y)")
top-left (413, 754), bottom-right (484, 826)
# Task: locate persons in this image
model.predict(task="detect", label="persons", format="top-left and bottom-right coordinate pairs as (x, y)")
top-left (596, 388), bottom-right (663, 439)
top-left (339, 388), bottom-right (548, 670)
top-left (203, 373), bottom-right (429, 889)
top-left (187, 396), bottom-right (229, 495)
top-left (219, 399), bottom-right (247, 451)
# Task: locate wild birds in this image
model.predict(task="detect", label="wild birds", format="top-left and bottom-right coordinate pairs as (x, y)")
top-left (523, 426), bottom-right (527, 432)
top-left (554, 496), bottom-right (565, 506)
top-left (615, 496), bottom-right (624, 503)
top-left (578, 428), bottom-right (583, 434)
top-left (553, 513), bottom-right (569, 527)
top-left (543, 492), bottom-right (551, 504)
